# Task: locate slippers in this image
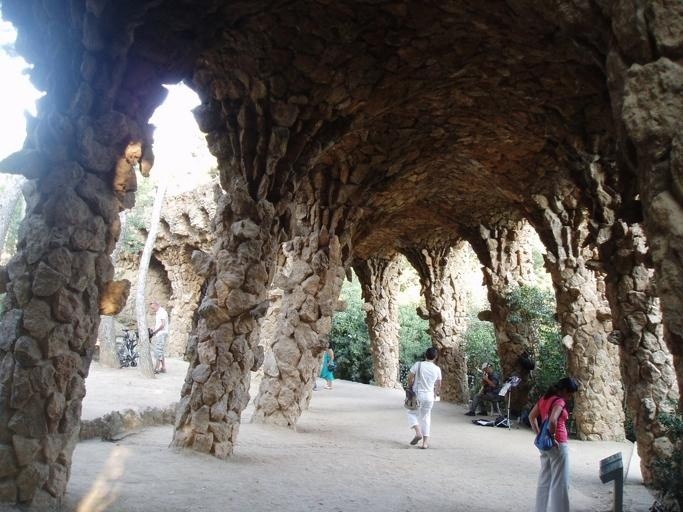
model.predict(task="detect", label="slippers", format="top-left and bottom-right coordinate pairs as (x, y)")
top-left (408, 434), bottom-right (430, 449)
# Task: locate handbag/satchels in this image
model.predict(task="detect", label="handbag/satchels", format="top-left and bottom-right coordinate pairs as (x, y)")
top-left (327, 363), bottom-right (337, 372)
top-left (402, 386), bottom-right (419, 410)
top-left (533, 417), bottom-right (555, 451)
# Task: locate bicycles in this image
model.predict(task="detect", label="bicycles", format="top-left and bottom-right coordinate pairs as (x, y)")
top-left (115, 327), bottom-right (155, 369)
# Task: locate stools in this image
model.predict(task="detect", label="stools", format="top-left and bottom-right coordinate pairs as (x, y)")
top-left (488, 398), bottom-right (504, 416)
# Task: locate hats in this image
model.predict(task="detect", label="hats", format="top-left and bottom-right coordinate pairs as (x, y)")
top-left (480, 362), bottom-right (490, 370)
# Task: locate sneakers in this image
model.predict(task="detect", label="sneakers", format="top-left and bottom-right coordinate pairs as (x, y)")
top-left (463, 410), bottom-right (489, 416)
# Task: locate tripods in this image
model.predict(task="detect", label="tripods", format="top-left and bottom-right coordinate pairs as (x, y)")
top-left (496, 386), bottom-right (519, 430)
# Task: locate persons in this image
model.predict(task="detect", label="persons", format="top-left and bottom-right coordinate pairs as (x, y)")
top-left (404, 348), bottom-right (442, 449)
top-left (462, 361), bottom-right (500, 417)
top-left (528, 376), bottom-right (578, 511)
top-left (319, 337), bottom-right (335, 390)
top-left (147, 298), bottom-right (169, 373)
